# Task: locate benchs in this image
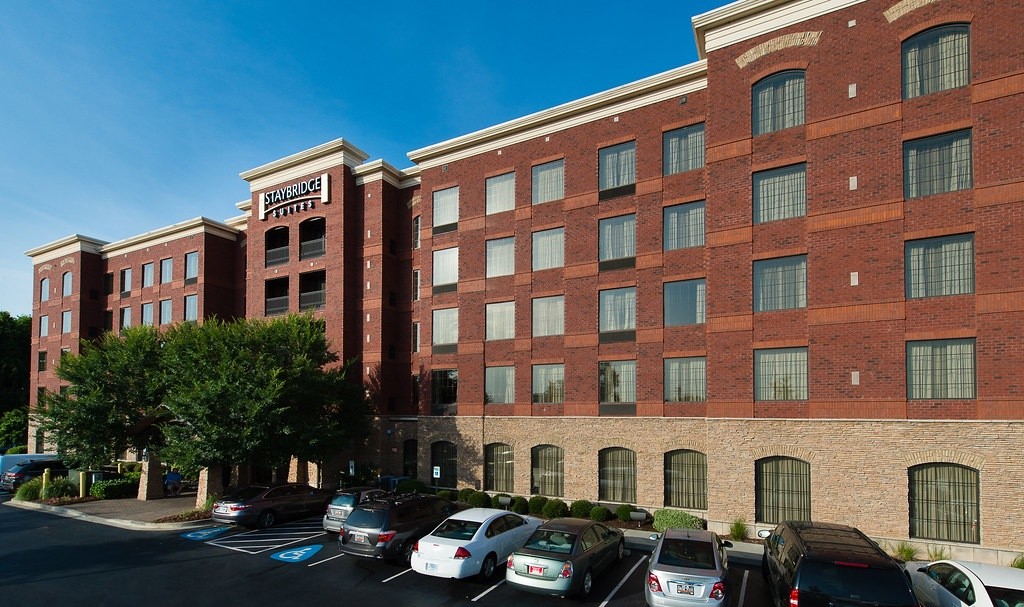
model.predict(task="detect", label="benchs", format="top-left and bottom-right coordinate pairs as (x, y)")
top-left (164, 483), bottom-right (198, 498)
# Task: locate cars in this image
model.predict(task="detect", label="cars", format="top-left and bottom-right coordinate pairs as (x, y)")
top-left (905, 559), bottom-right (1024, 607)
top-left (211, 482), bottom-right (336, 530)
top-left (506, 518), bottom-right (625, 599)
top-left (410, 507), bottom-right (544, 580)
top-left (644, 527), bottom-right (733, 607)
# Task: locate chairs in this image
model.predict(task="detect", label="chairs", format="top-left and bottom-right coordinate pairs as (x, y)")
top-left (995, 599), bottom-right (1024, 607)
top-left (538, 541), bottom-right (572, 548)
top-left (550, 535), bottom-right (566, 545)
top-left (956, 588), bottom-right (974, 601)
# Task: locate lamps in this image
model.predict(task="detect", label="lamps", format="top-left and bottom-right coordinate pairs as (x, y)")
top-left (630, 512), bottom-right (646, 528)
top-left (499, 496), bottom-right (511, 511)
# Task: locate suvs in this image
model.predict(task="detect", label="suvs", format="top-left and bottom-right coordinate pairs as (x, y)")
top-left (338, 491), bottom-right (475, 568)
top-left (322, 487), bottom-right (390, 534)
top-left (757, 520), bottom-right (921, 607)
top-left (0, 460), bottom-right (69, 494)
